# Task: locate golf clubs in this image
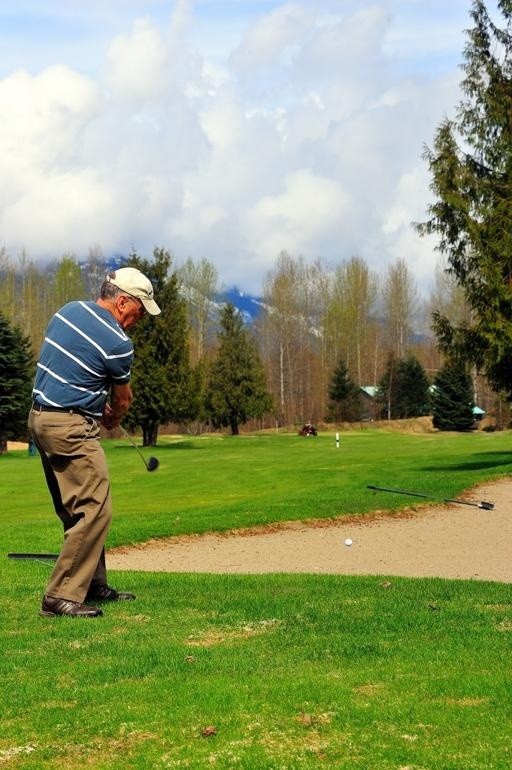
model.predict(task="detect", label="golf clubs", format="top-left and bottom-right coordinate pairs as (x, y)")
top-left (119, 425), bottom-right (159, 472)
top-left (367, 485), bottom-right (494, 510)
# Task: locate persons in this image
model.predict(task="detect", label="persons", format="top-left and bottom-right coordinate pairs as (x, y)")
top-left (27, 267), bottom-right (162, 617)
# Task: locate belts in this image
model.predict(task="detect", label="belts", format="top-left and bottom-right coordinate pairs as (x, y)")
top-left (29, 399), bottom-right (85, 416)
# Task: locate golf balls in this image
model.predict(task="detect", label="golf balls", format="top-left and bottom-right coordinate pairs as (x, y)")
top-left (345, 537), bottom-right (353, 547)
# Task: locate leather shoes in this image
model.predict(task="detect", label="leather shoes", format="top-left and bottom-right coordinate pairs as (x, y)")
top-left (87, 584), bottom-right (136, 603)
top-left (39, 595), bottom-right (101, 617)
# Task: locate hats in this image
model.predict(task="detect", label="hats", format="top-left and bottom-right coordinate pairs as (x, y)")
top-left (103, 264), bottom-right (163, 318)
